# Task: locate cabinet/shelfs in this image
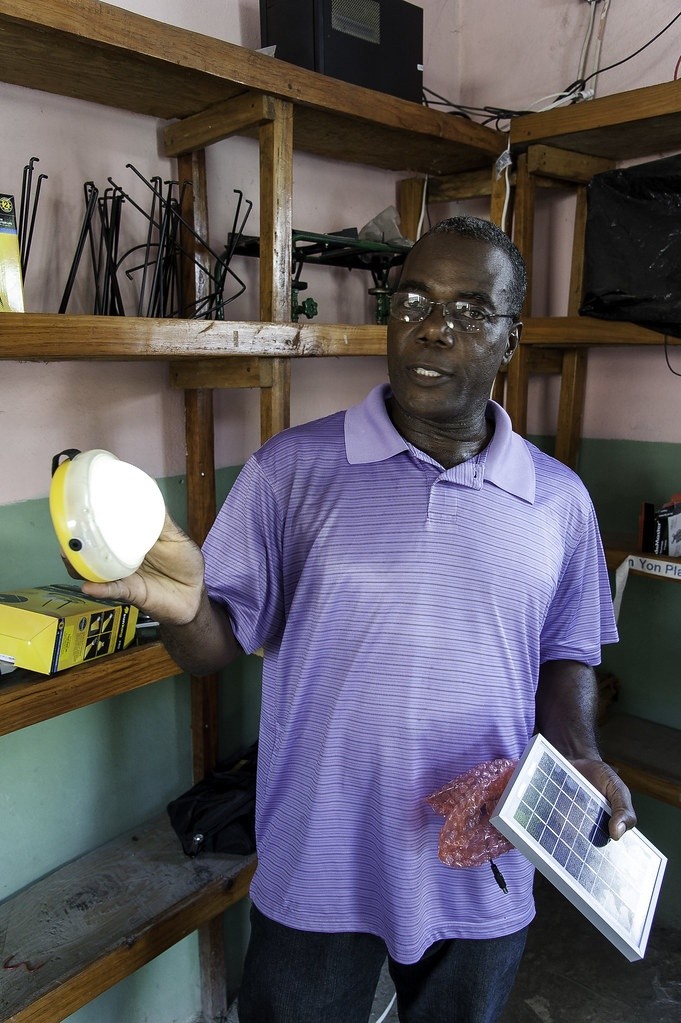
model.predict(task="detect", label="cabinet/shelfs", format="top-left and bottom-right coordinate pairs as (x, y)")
top-left (0, 0), bottom-right (680, 1023)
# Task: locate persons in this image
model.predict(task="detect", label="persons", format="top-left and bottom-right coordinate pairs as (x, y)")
top-left (60, 216), bottom-right (637, 1022)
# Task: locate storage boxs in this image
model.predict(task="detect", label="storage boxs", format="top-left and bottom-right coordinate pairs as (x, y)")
top-left (0, 584), bottom-right (140, 677)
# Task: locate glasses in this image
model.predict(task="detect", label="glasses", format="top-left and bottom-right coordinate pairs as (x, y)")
top-left (385, 291), bottom-right (519, 335)
